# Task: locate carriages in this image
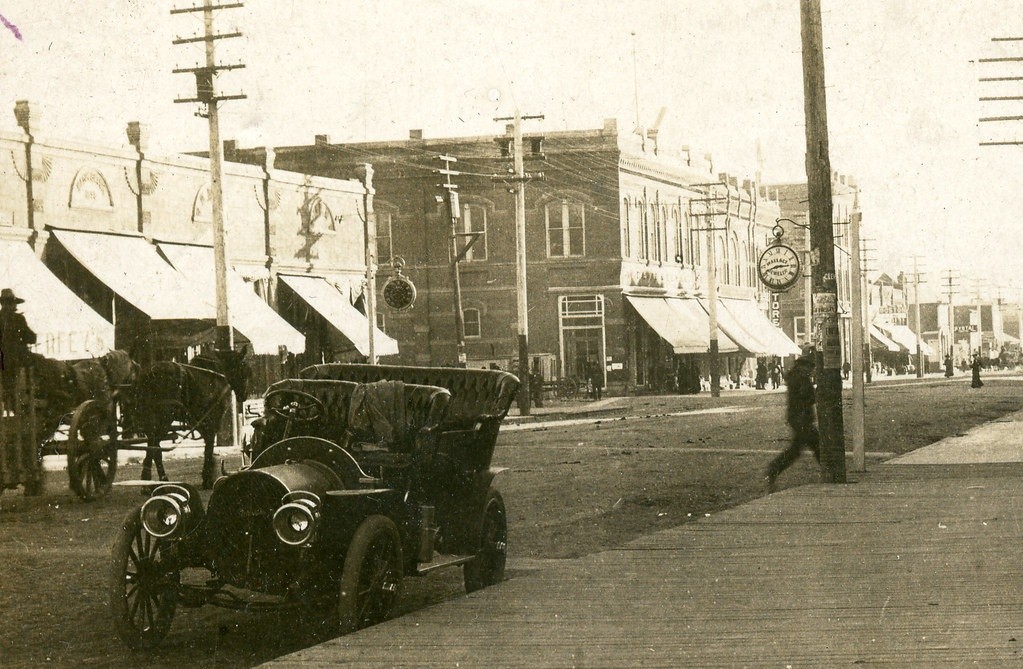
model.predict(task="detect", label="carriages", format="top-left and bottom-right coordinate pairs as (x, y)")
top-left (1, 339), bottom-right (251, 505)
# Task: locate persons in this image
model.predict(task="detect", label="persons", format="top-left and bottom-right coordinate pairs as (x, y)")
top-left (590, 361), bottom-right (603, 402)
top-left (944, 354), bottom-right (954, 378)
top-left (0, 289), bottom-right (37, 353)
top-left (766, 342), bottom-right (821, 486)
top-left (756, 360), bottom-right (780, 390)
top-left (843, 361), bottom-right (850, 380)
top-left (961, 345), bottom-right (1023, 388)
top-left (678, 361), bottom-right (702, 394)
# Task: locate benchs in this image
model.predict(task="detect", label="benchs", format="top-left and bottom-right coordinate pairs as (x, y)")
top-left (298, 363), bottom-right (523, 473)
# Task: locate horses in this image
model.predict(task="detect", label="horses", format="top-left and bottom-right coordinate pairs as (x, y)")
top-left (46, 344), bottom-right (253, 493)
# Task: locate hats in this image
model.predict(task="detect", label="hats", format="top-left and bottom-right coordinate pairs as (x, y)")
top-left (0, 289), bottom-right (25, 303)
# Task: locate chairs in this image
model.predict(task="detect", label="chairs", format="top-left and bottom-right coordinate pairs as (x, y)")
top-left (343, 379), bottom-right (454, 487)
top-left (261, 379), bottom-right (358, 452)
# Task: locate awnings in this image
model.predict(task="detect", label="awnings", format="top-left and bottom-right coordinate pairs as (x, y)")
top-left (698, 300), bottom-right (776, 357)
top-left (0, 240), bottom-right (116, 359)
top-left (875, 324), bottom-right (936, 355)
top-left (626, 296), bottom-right (709, 353)
top-left (665, 297), bottom-right (739, 353)
top-left (869, 325), bottom-right (900, 351)
top-left (720, 300), bottom-right (802, 356)
top-left (278, 273), bottom-right (399, 356)
top-left (52, 230), bottom-right (219, 321)
top-left (154, 241), bottom-right (305, 357)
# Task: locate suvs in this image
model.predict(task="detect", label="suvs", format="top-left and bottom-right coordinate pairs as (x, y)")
top-left (109, 361), bottom-right (523, 655)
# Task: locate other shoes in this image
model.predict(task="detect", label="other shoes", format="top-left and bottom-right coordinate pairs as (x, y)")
top-left (762, 470), bottom-right (775, 492)
